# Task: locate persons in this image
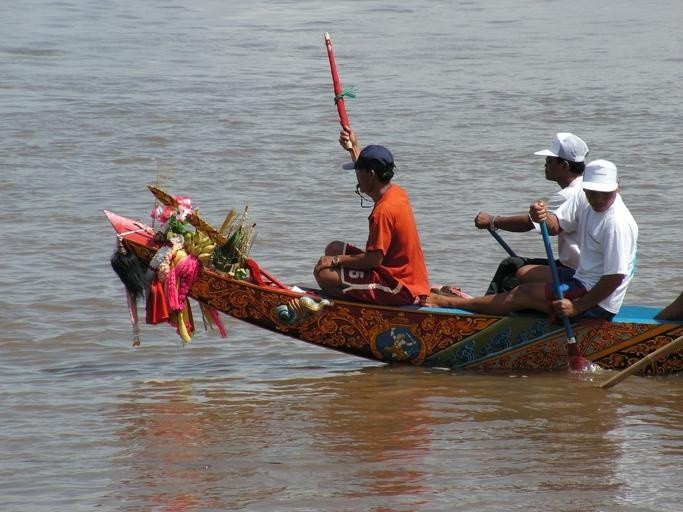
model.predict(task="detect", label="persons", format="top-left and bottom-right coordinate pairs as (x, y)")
top-left (312, 123), bottom-right (430, 309)
top-left (471, 129), bottom-right (591, 298)
top-left (419, 157), bottom-right (640, 325)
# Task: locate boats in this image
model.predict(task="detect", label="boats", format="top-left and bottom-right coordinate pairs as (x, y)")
top-left (99, 202), bottom-right (683, 381)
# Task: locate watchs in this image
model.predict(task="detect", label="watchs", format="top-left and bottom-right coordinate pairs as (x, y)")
top-left (332, 254), bottom-right (340, 267)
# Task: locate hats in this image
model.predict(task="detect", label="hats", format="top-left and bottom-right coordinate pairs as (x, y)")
top-left (533, 132), bottom-right (589, 163)
top-left (582, 159), bottom-right (619, 193)
top-left (342, 145), bottom-right (394, 171)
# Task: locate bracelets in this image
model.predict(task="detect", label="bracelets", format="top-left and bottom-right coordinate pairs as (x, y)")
top-left (489, 214), bottom-right (499, 231)
